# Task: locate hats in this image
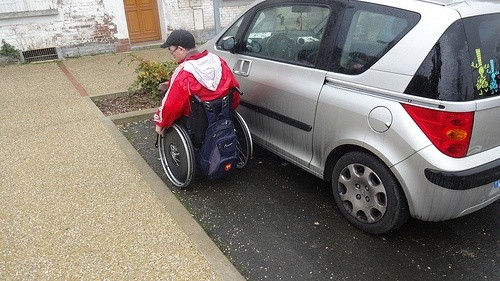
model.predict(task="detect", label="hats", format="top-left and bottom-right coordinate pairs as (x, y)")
top-left (160, 30), bottom-right (195, 48)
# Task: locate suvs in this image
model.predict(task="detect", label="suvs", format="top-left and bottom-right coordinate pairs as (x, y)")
top-left (190, 0), bottom-right (500, 236)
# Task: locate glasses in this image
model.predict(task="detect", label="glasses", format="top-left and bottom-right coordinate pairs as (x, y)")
top-left (169, 47), bottom-right (178, 55)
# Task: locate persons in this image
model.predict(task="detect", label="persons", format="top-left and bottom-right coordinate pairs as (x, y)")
top-left (155, 30), bottom-right (241, 135)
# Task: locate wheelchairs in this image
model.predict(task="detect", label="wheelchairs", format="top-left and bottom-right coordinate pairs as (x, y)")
top-left (155, 86), bottom-right (254, 189)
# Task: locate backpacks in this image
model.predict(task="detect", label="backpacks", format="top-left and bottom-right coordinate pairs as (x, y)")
top-left (199, 97), bottom-right (238, 179)
